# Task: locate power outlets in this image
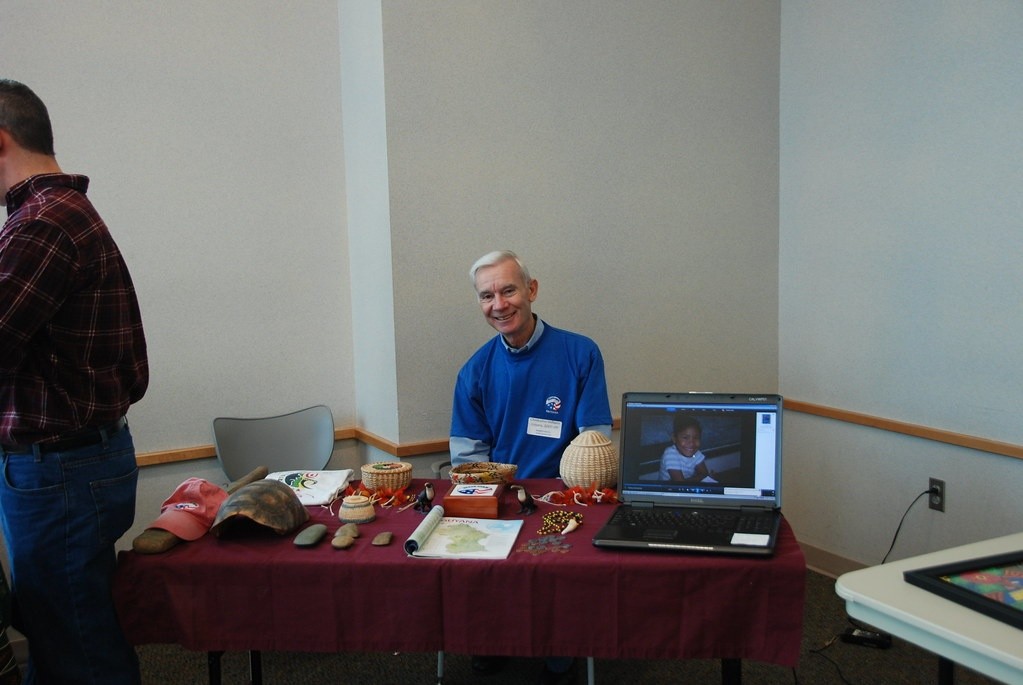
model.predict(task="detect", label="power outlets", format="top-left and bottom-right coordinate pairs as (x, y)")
top-left (928, 476), bottom-right (946, 512)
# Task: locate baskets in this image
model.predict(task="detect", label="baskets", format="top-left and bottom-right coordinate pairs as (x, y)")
top-left (361, 461), bottom-right (412, 492)
top-left (559, 430), bottom-right (618, 492)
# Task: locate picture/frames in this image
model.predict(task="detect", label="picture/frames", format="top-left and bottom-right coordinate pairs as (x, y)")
top-left (903, 549), bottom-right (1023, 637)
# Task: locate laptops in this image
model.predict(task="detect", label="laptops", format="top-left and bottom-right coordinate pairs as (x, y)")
top-left (593, 392), bottom-right (783, 559)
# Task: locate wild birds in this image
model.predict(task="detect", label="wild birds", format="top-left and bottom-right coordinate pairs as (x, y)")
top-left (413, 481), bottom-right (435, 514)
top-left (510, 483), bottom-right (538, 515)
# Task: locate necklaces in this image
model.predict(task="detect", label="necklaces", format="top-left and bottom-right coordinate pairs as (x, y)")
top-left (531, 507), bottom-right (584, 535)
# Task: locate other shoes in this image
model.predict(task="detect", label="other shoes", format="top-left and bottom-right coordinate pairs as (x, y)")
top-left (538, 657), bottom-right (579, 685)
top-left (472, 655), bottom-right (511, 674)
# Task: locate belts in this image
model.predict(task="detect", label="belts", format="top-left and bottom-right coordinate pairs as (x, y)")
top-left (0, 416), bottom-right (125, 454)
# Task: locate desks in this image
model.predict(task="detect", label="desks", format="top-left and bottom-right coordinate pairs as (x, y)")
top-left (834, 531), bottom-right (1023, 685)
top-left (116, 480), bottom-right (806, 685)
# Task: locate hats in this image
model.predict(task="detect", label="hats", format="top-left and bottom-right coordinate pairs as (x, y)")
top-left (144, 477), bottom-right (229, 540)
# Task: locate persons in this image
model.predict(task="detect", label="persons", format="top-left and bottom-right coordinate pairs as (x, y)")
top-left (0, 77), bottom-right (150, 685)
top-left (658, 415), bottom-right (718, 483)
top-left (447, 250), bottom-right (613, 684)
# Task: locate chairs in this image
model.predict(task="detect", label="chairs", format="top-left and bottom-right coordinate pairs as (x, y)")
top-left (212, 403), bottom-right (335, 684)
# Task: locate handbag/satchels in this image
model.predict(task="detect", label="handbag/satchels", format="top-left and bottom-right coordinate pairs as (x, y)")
top-left (264, 469), bottom-right (354, 509)
